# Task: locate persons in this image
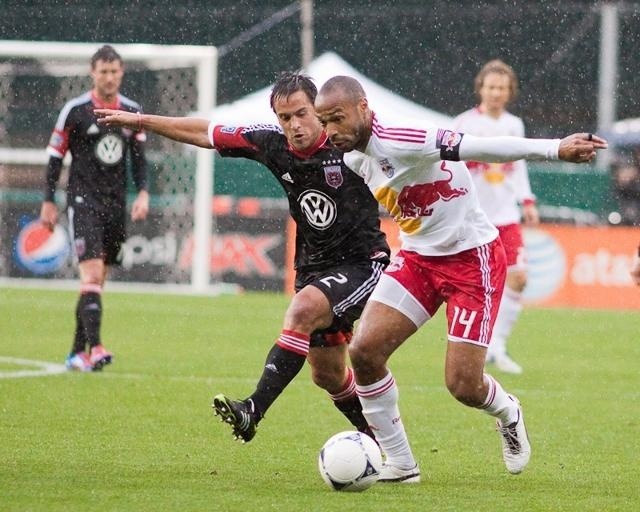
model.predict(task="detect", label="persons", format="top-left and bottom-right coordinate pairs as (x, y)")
top-left (92, 76), bottom-right (379, 446)
top-left (312, 76), bottom-right (608, 485)
top-left (450, 58), bottom-right (541, 375)
top-left (39, 46), bottom-right (149, 373)
top-left (606, 156), bottom-right (640, 226)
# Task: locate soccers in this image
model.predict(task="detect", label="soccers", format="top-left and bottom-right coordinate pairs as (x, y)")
top-left (318, 430), bottom-right (382, 493)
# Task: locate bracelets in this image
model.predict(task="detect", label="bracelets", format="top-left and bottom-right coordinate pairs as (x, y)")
top-left (135, 110), bottom-right (143, 132)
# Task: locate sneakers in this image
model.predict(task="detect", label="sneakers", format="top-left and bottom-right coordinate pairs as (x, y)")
top-left (212, 392), bottom-right (258, 443)
top-left (379, 464), bottom-right (422, 483)
top-left (496, 394), bottom-right (531, 474)
top-left (64, 346), bottom-right (111, 372)
